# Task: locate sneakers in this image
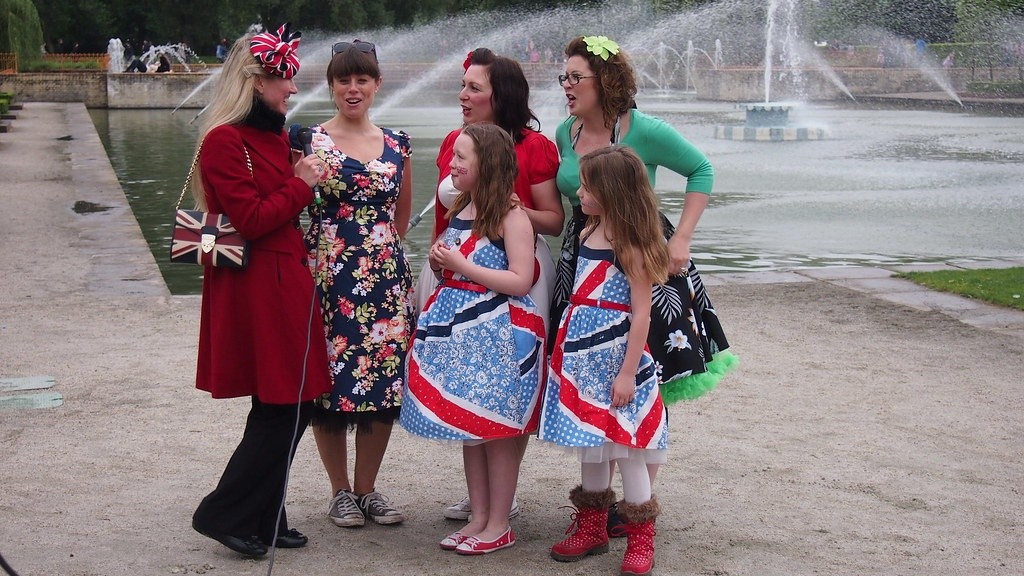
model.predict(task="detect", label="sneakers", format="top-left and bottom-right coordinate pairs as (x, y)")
top-left (327, 489), bottom-right (365, 527)
top-left (352, 488), bottom-right (404, 524)
top-left (444, 495), bottom-right (519, 521)
top-left (440, 526), bottom-right (516, 554)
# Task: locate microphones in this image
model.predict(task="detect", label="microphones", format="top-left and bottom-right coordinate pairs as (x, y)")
top-left (297, 127), bottom-right (323, 203)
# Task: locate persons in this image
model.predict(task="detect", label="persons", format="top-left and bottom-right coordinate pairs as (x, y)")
top-left (554, 35), bottom-right (736, 536)
top-left (192, 21), bottom-right (332, 556)
top-left (216, 38), bottom-right (230, 63)
top-left (288, 38), bottom-right (419, 527)
top-left (817, 36), bottom-right (956, 67)
top-left (124, 38), bottom-right (170, 74)
top-left (517, 40), bottom-right (566, 63)
top-left (396, 120), bottom-right (548, 555)
top-left (40, 38), bottom-right (81, 53)
top-left (536, 145), bottom-right (672, 576)
top-left (415, 48), bottom-right (565, 522)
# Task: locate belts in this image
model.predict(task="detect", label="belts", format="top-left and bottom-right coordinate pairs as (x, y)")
top-left (570, 295), bottom-right (631, 312)
top-left (444, 280), bottom-right (486, 292)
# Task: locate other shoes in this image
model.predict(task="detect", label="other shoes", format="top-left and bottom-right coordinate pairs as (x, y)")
top-left (607, 502), bottom-right (627, 537)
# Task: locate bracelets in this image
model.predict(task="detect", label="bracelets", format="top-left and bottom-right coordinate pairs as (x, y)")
top-left (432, 266), bottom-right (441, 272)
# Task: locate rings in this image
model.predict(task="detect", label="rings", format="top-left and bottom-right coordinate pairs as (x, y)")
top-left (680, 266), bottom-right (686, 272)
top-left (317, 165), bottom-right (322, 171)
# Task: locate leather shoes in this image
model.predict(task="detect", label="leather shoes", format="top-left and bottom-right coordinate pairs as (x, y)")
top-left (254, 528), bottom-right (308, 548)
top-left (192, 520), bottom-right (268, 555)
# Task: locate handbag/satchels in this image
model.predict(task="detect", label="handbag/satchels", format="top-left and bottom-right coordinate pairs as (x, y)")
top-left (170, 122), bottom-right (253, 271)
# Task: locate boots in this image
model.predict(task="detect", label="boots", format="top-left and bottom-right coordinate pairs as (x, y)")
top-left (550, 484), bottom-right (616, 561)
top-left (617, 494), bottom-right (660, 576)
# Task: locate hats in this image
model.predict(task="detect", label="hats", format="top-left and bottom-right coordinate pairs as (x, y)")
top-left (250, 22), bottom-right (301, 78)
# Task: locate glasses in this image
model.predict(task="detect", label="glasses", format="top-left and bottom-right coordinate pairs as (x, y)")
top-left (332, 42), bottom-right (378, 63)
top-left (559, 74), bottom-right (598, 86)
